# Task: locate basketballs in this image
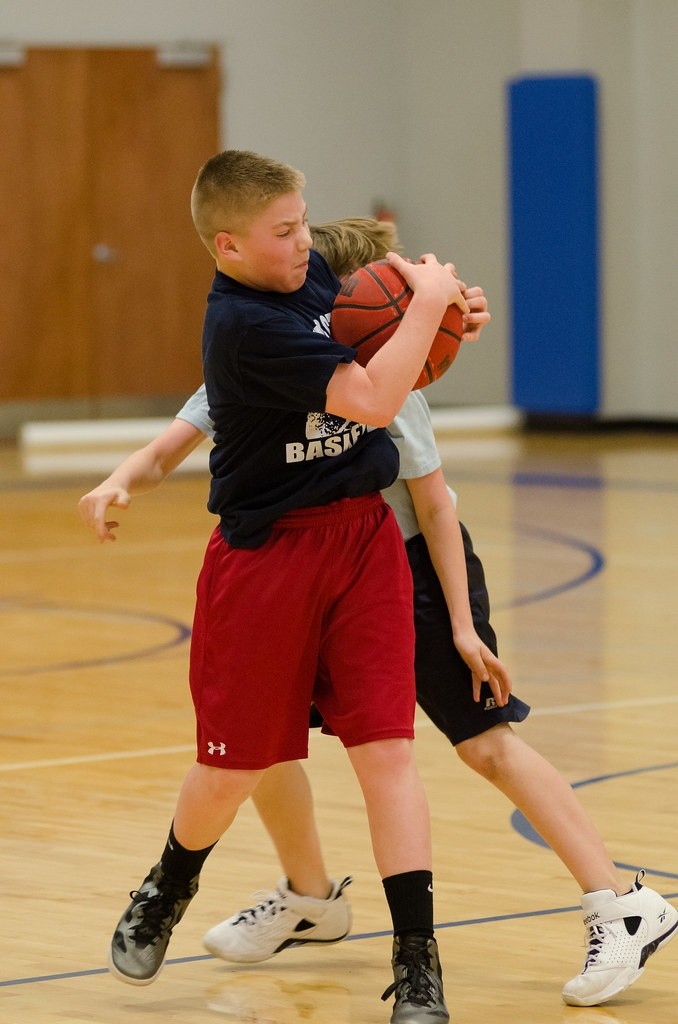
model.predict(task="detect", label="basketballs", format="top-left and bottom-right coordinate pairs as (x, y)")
top-left (331, 257), bottom-right (465, 393)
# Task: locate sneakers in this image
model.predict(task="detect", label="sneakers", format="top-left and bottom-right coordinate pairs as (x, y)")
top-left (108, 859), bottom-right (199, 986)
top-left (380, 934), bottom-right (450, 1024)
top-left (560, 868), bottom-right (678, 1007)
top-left (204, 875), bottom-right (354, 964)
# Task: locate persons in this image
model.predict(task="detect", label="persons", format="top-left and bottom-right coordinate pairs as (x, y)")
top-left (109, 152), bottom-right (491, 1024)
top-left (81, 219), bottom-right (677, 1007)
